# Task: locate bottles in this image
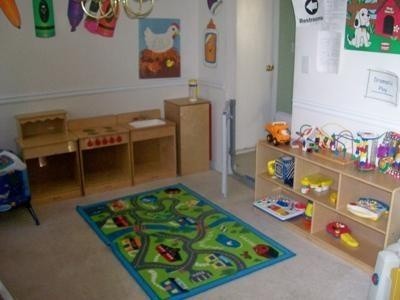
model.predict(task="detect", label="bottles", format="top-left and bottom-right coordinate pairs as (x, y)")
top-left (189, 79), bottom-right (198, 103)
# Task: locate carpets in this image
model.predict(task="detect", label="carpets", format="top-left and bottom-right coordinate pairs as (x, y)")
top-left (76, 182), bottom-right (296, 300)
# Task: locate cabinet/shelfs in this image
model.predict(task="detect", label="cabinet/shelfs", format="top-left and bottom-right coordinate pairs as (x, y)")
top-left (13, 96), bottom-right (212, 206)
top-left (254, 137), bottom-right (400, 279)
